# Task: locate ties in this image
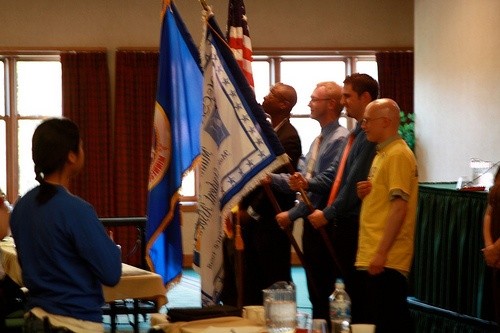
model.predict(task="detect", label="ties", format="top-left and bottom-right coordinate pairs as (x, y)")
top-left (305, 132), bottom-right (324, 180)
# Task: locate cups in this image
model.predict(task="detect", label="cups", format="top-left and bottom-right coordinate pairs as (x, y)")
top-left (312, 320), bottom-right (326, 333)
top-left (351, 324), bottom-right (376, 333)
top-left (243, 305), bottom-right (265, 326)
top-left (296, 313), bottom-right (308, 333)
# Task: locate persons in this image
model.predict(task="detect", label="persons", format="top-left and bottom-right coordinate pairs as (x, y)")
top-left (288, 73), bottom-right (379, 325)
top-left (481, 166), bottom-right (500, 333)
top-left (266, 81), bottom-right (351, 318)
top-left (233, 83), bottom-right (298, 307)
top-left (8, 118), bottom-right (122, 333)
top-left (354, 98), bottom-right (417, 333)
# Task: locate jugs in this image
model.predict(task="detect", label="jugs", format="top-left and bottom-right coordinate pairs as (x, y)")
top-left (471, 159), bottom-right (493, 190)
top-left (263, 281), bottom-right (297, 333)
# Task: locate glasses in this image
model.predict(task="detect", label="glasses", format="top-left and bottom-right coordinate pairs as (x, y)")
top-left (361, 117), bottom-right (386, 123)
top-left (310, 96), bottom-right (335, 102)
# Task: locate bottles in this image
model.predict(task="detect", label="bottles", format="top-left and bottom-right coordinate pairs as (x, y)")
top-left (329, 279), bottom-right (350, 333)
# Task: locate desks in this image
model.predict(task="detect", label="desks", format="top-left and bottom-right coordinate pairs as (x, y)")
top-left (408, 182), bottom-right (500, 333)
top-left (0, 236), bottom-right (168, 333)
top-left (180, 314), bottom-right (263, 333)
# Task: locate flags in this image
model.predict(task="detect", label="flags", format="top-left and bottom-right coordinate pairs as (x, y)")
top-left (146, 0), bottom-right (204, 292)
top-left (223, 0), bottom-right (256, 239)
top-left (198, 0), bottom-right (290, 307)
